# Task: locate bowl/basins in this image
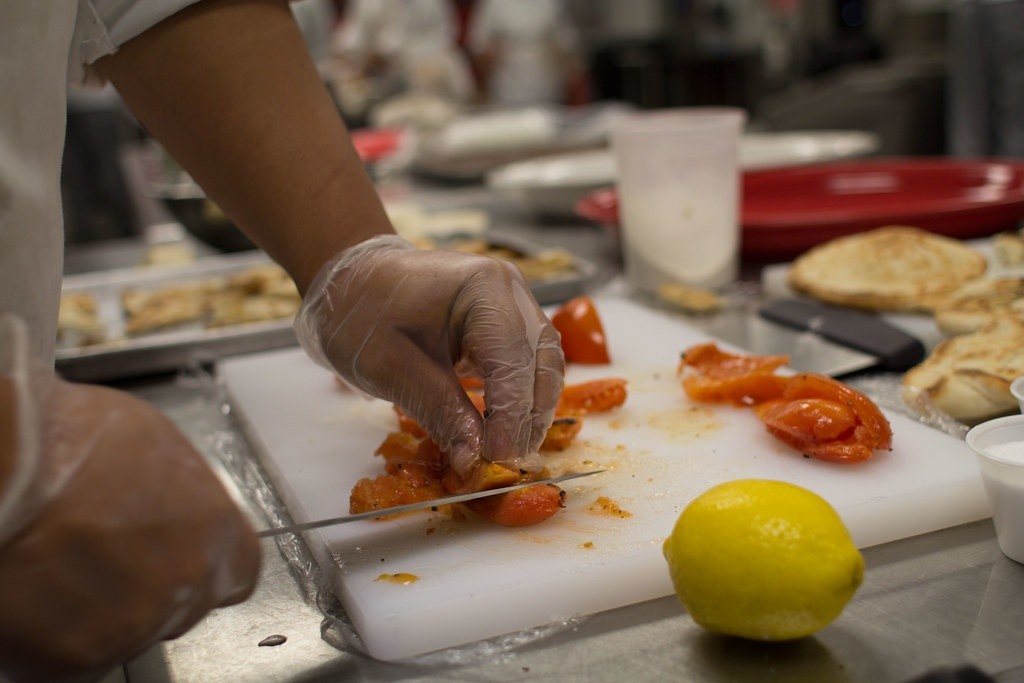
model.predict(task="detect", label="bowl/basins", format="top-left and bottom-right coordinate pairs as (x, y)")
top-left (964, 375), bottom-right (1024, 564)
top-left (150, 166), bottom-right (395, 255)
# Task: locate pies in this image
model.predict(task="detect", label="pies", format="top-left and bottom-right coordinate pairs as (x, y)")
top-left (786, 227), bottom-right (1024, 423)
top-left (57, 236), bottom-right (573, 347)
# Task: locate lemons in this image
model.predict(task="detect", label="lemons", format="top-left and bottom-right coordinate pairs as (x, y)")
top-left (662, 478), bottom-right (865, 643)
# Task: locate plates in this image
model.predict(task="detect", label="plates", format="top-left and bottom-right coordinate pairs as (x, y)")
top-left (577, 158), bottom-right (1024, 263)
top-left (486, 131), bottom-right (879, 223)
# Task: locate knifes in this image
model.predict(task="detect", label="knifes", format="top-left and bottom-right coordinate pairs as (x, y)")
top-left (257, 469), bottom-right (607, 538)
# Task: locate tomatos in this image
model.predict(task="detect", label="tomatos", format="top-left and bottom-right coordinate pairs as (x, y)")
top-left (350, 295), bottom-right (894, 528)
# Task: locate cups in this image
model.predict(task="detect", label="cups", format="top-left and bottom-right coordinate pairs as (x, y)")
top-left (607, 107), bottom-right (743, 292)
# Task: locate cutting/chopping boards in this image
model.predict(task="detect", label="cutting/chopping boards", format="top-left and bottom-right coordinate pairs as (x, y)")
top-left (217, 298), bottom-right (994, 658)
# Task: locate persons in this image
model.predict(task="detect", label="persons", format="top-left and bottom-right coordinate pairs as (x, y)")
top-left (328, 1), bottom-right (587, 110)
top-left (1, 1), bottom-right (565, 683)
